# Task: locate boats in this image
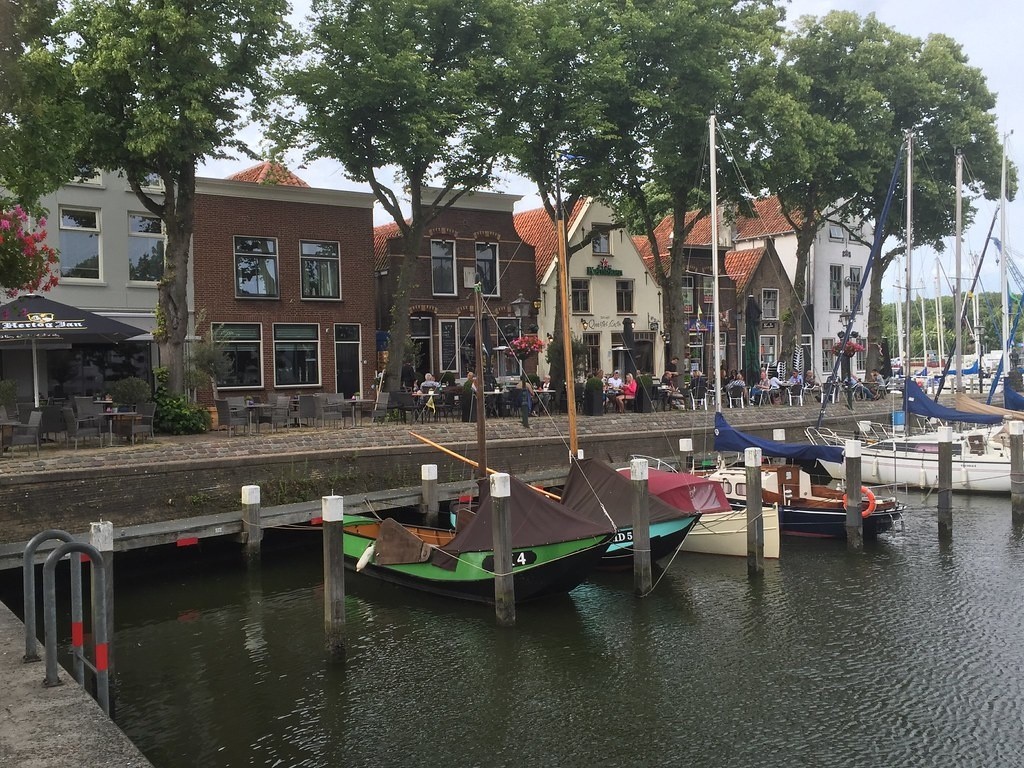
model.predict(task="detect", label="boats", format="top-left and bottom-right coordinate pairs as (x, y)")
top-left (616, 466), bottom-right (784, 559)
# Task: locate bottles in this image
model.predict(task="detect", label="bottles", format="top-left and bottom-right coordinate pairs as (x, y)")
top-left (438, 385), bottom-right (443, 393)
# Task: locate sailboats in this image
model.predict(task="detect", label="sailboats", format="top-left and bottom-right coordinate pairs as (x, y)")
top-left (342, 285), bottom-right (616, 606)
top-left (805, 134), bottom-right (1012, 495)
top-left (542, 224), bottom-right (702, 569)
top-left (697, 113), bottom-right (906, 542)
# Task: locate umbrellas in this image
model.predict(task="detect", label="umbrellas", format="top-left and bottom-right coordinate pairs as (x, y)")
top-left (0, 294), bottom-right (150, 406)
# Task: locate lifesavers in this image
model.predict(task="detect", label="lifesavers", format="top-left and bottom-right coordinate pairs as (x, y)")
top-left (843, 485), bottom-right (877, 519)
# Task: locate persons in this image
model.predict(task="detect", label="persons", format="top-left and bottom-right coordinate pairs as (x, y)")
top-left (411, 355), bottom-right (885, 414)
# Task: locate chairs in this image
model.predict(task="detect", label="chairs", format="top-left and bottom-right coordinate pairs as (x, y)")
top-left (601, 375), bottom-right (898, 414)
top-left (0, 394), bottom-right (157, 461)
top-left (214, 381), bottom-right (586, 438)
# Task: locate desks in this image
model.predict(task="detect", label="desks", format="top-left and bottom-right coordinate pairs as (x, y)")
top-left (533, 389), bottom-right (556, 417)
top-left (779, 384), bottom-right (792, 407)
top-left (603, 388), bottom-right (622, 413)
top-left (345, 400), bottom-right (373, 429)
top-left (241, 403), bottom-right (274, 438)
top-left (98, 412), bottom-right (138, 448)
top-left (412, 393), bottom-right (439, 423)
top-left (0, 422), bottom-right (23, 458)
top-left (485, 391), bottom-right (503, 418)
top-left (863, 381), bottom-right (880, 399)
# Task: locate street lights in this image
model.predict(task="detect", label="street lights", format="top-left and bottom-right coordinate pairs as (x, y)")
top-left (837, 305), bottom-right (854, 410)
top-left (974, 318), bottom-right (984, 393)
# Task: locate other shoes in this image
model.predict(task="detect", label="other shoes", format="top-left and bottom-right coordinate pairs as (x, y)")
top-left (874, 394), bottom-right (880, 400)
